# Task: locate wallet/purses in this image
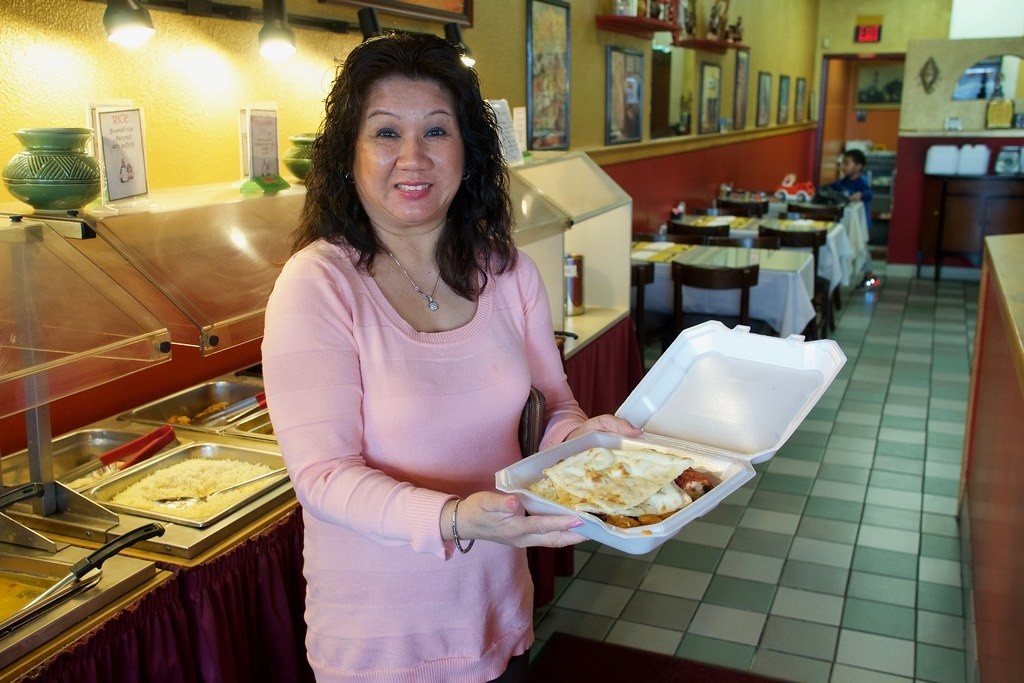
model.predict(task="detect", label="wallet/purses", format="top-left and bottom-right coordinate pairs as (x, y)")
top-left (518, 386), bottom-right (546, 459)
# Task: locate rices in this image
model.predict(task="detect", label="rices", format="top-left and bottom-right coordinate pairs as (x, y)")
top-left (529, 478), bottom-right (561, 504)
top-left (109, 459), bottom-right (285, 519)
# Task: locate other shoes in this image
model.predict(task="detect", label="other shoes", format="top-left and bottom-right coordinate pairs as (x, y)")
top-left (861, 272), bottom-right (879, 289)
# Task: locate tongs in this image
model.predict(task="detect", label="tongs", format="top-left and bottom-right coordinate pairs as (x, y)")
top-left (188, 391), bottom-right (267, 427)
top-left (54, 425), bottom-right (177, 494)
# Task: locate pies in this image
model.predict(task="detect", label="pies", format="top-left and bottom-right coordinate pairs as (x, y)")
top-left (543, 447), bottom-right (695, 516)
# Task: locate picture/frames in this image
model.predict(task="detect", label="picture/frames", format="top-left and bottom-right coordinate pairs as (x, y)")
top-left (757, 72), bottom-right (771, 126)
top-left (526, 0), bottom-right (571, 151)
top-left (698, 62), bottom-right (721, 134)
top-left (777, 75), bottom-right (790, 124)
top-left (793, 78), bottom-right (806, 121)
top-left (733, 48), bottom-right (749, 129)
top-left (605, 45), bottom-right (643, 145)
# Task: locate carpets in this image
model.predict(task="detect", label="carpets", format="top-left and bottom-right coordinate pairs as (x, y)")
top-left (524, 631), bottom-right (781, 683)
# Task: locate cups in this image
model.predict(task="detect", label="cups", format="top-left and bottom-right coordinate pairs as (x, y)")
top-left (562, 254), bottom-right (586, 316)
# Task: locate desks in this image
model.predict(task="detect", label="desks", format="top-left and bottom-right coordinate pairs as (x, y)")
top-left (710, 195), bottom-right (869, 311)
top-left (630, 241), bottom-right (816, 341)
top-left (655, 214), bottom-right (853, 340)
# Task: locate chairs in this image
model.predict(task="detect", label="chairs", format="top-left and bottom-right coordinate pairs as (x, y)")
top-left (632, 200), bottom-right (844, 356)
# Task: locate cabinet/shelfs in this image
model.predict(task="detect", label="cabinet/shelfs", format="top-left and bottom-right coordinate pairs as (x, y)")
top-left (917, 176), bottom-right (1024, 282)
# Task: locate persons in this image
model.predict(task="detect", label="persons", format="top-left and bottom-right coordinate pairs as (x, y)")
top-left (818, 150), bottom-right (877, 289)
top-left (262, 32), bottom-right (644, 683)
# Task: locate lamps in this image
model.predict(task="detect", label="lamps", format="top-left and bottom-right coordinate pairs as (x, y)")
top-left (259, 0), bottom-right (297, 59)
top-left (101, 0), bottom-right (153, 45)
top-left (356, 6), bottom-right (385, 43)
top-left (444, 22), bottom-right (476, 68)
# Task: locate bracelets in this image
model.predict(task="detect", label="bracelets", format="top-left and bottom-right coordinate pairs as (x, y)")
top-left (452, 499), bottom-right (474, 555)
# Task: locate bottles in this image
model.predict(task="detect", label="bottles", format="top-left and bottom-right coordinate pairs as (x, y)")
top-left (280, 133), bottom-right (321, 180)
top-left (2, 127), bottom-right (102, 216)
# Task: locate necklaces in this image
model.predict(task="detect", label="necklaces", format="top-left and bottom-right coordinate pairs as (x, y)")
top-left (386, 247), bottom-right (440, 310)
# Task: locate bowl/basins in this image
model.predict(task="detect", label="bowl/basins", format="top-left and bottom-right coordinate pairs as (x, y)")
top-left (493, 319), bottom-right (848, 555)
